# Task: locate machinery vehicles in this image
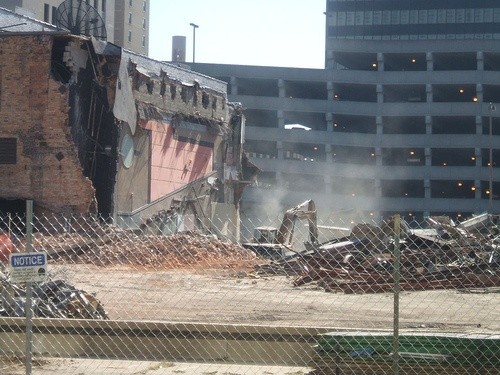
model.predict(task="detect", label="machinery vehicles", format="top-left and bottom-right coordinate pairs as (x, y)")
top-left (243, 198), bottom-right (319, 258)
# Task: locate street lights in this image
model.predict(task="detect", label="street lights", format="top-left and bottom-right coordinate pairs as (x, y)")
top-left (190, 22), bottom-right (198, 62)
top-left (487, 102), bottom-right (497, 211)
